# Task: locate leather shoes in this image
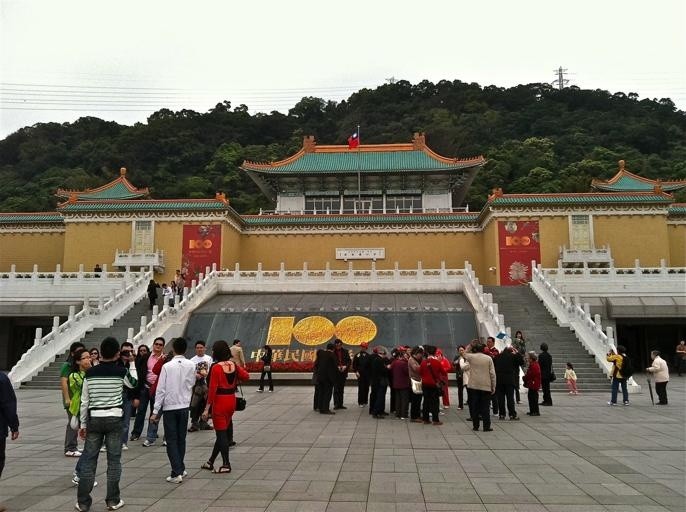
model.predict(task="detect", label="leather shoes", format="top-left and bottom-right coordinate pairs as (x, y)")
top-left (312, 400), bottom-right (554, 432)
top-left (656, 402), bottom-right (666, 405)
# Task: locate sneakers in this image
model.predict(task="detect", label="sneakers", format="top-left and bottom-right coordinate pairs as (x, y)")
top-left (130, 435), bottom-right (139, 441)
top-left (75, 502), bottom-right (82, 512)
top-left (166, 474), bottom-right (182, 484)
top-left (187, 423), bottom-right (200, 432)
top-left (65, 448), bottom-right (83, 457)
top-left (228, 441), bottom-right (236, 446)
top-left (199, 421), bottom-right (214, 430)
top-left (256, 390), bottom-right (263, 393)
top-left (575, 391), bottom-right (577, 395)
top-left (122, 443), bottom-right (129, 450)
top-left (107, 499), bottom-right (125, 510)
top-left (269, 391), bottom-right (272, 393)
top-left (181, 470), bottom-right (187, 477)
top-left (100, 446), bottom-right (107, 452)
top-left (608, 401), bottom-right (618, 405)
top-left (71, 470), bottom-right (98, 487)
top-left (570, 391), bottom-right (573, 394)
top-left (625, 400), bottom-right (630, 405)
top-left (160, 441), bottom-right (167, 446)
top-left (142, 440), bottom-right (155, 447)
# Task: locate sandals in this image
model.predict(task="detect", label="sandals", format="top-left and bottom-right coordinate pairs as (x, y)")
top-left (200, 461), bottom-right (214, 470)
top-left (211, 465), bottom-right (231, 473)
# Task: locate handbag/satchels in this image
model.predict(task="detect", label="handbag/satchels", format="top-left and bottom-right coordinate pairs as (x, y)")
top-left (551, 372), bottom-right (556, 382)
top-left (263, 365), bottom-right (271, 372)
top-left (69, 415), bottom-right (79, 430)
top-left (411, 378), bottom-right (424, 395)
top-left (436, 382), bottom-right (444, 396)
top-left (235, 396), bottom-right (247, 411)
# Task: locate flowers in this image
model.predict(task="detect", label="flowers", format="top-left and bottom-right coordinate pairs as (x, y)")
top-left (246, 360), bottom-right (315, 372)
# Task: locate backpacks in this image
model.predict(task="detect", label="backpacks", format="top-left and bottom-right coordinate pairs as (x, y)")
top-left (618, 355), bottom-right (632, 379)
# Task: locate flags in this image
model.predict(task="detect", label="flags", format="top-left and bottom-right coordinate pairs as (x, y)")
top-left (348, 132), bottom-right (359, 149)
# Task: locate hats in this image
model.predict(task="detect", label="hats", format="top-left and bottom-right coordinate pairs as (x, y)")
top-left (361, 341), bottom-right (368, 348)
top-left (435, 348), bottom-right (442, 357)
top-left (399, 345), bottom-right (407, 351)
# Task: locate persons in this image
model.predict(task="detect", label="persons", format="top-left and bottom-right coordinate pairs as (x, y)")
top-left (676, 339), bottom-right (686, 376)
top-left (607, 345), bottom-right (630, 405)
top-left (0, 371), bottom-right (19, 512)
top-left (256, 345), bottom-right (274, 393)
top-left (645, 349), bottom-right (669, 405)
top-left (564, 362), bottom-right (578, 394)
top-left (311, 331), bottom-right (552, 432)
top-left (61, 336), bottom-right (250, 512)
top-left (94, 264), bottom-right (102, 278)
top-left (147, 263), bottom-right (198, 309)
